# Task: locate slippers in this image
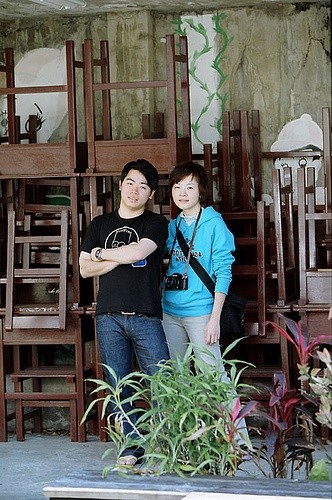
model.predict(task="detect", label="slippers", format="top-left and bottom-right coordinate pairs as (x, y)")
top-left (117, 454), bottom-right (138, 465)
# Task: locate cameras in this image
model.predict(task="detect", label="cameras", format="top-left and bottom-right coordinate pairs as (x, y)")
top-left (165, 273), bottom-right (188, 291)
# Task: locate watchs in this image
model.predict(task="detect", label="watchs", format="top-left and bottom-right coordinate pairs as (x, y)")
top-left (95, 247), bottom-right (105, 261)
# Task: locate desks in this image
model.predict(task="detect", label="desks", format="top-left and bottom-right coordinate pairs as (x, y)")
top-left (0, 32), bottom-right (332, 445)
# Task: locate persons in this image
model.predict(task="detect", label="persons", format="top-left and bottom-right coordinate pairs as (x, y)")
top-left (78, 158), bottom-right (189, 468)
top-left (159, 162), bottom-right (253, 462)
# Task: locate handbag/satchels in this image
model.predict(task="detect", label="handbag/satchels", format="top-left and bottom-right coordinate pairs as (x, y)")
top-left (218, 291), bottom-right (247, 343)
